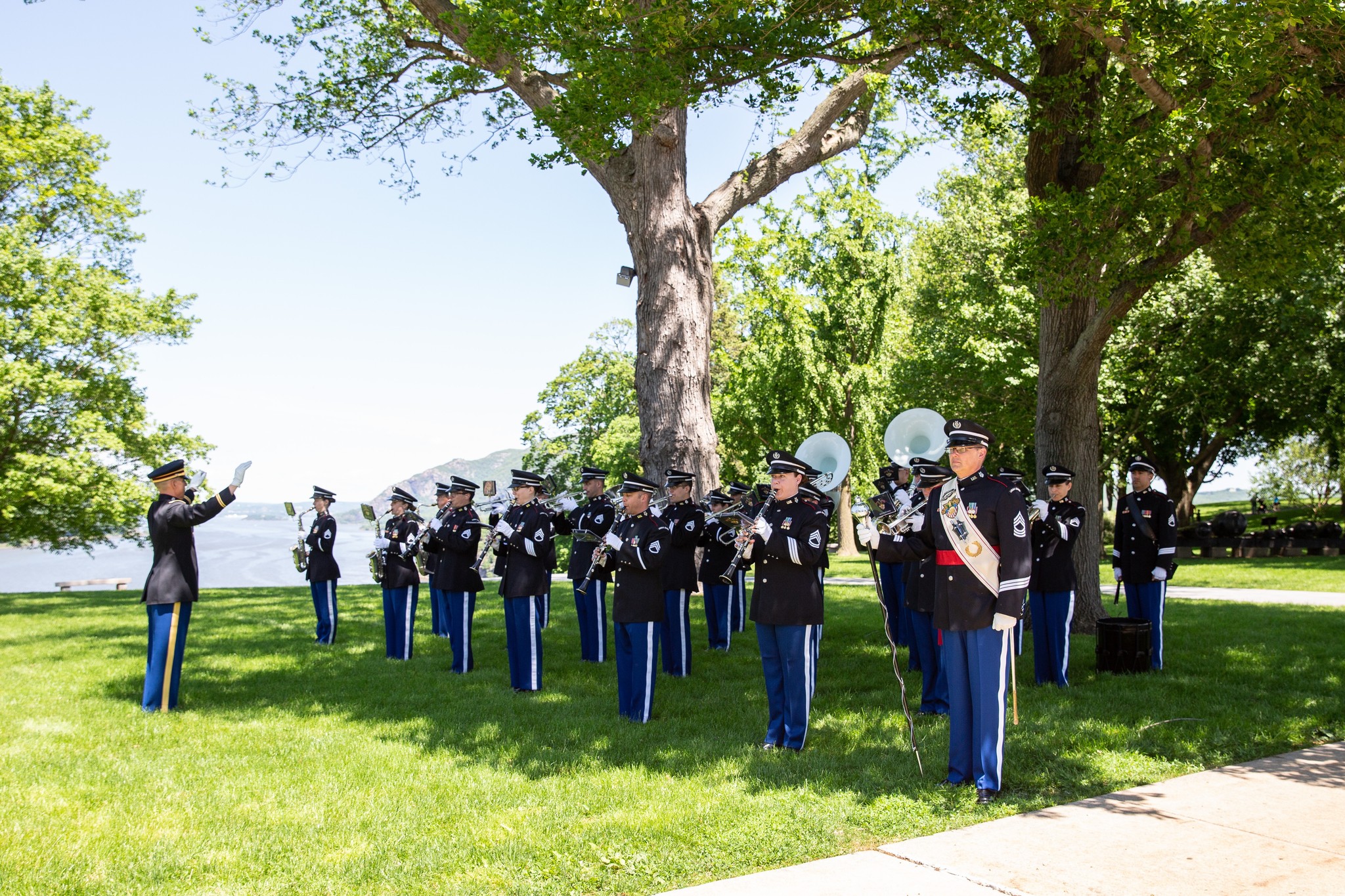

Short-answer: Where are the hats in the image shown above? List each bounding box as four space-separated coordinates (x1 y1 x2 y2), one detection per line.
309 485 337 499
706 481 751 505
997 467 1027 482
909 457 941 475
888 457 903 468
916 465 956 488
766 450 826 502
1128 455 1160 475
944 418 996 448
1042 465 1076 485
387 468 552 504
148 459 191 482
578 466 696 493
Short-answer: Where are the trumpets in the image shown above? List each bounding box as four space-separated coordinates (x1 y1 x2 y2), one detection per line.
876 497 931 535
1028 493 1056 525
472 497 515 513
616 494 673 521
538 490 590 509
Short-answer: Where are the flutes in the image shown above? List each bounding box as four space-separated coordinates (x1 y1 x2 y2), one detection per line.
470 499 517 573
575 505 626 595
397 501 455 561
719 489 779 586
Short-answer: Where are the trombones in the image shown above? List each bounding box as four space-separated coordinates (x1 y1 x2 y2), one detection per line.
704 500 744 523
605 482 625 504
718 471 834 546
868 483 918 525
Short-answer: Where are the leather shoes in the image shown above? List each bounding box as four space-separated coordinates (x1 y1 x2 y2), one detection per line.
975 788 998 804
935 779 973 788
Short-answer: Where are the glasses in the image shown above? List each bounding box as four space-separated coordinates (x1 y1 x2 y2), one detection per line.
944 446 983 454
671 485 687 490
451 491 466 497
512 486 528 491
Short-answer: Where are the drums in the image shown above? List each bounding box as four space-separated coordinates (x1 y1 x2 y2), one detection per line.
1093 618 1154 673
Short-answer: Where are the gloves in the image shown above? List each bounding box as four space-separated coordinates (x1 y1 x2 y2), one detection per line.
888 480 897 490
490 494 508 515
556 490 579 512
992 612 1017 631
905 512 924 532
231 460 252 488
894 489 912 506
188 471 206 492
898 503 912 518
1031 499 1049 521
856 516 880 549
297 531 309 540
373 535 390 548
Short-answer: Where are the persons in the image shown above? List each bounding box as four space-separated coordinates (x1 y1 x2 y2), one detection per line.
1189 504 1201 523
874 454 1032 719
418 482 452 638
138 459 253 714
297 485 342 645
1028 465 1086 690
650 469 705 680
484 470 549 694
699 465 835 659
373 485 421 661
591 471 672 725
856 418 1031 804
732 451 828 756
1112 454 1178 670
1250 492 1281 515
552 466 614 666
415 475 481 676
488 483 558 631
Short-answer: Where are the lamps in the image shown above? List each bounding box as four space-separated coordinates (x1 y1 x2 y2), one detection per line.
616 267 636 286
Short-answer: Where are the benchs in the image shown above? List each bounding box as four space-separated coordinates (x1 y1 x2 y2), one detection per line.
1176 535 1344 557
54 577 132 592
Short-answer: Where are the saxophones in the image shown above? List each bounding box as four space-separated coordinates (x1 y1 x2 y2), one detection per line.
287 506 317 572
411 501 438 576
365 508 393 584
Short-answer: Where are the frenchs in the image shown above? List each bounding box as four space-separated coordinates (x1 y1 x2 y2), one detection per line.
883 407 950 469
790 431 852 493
